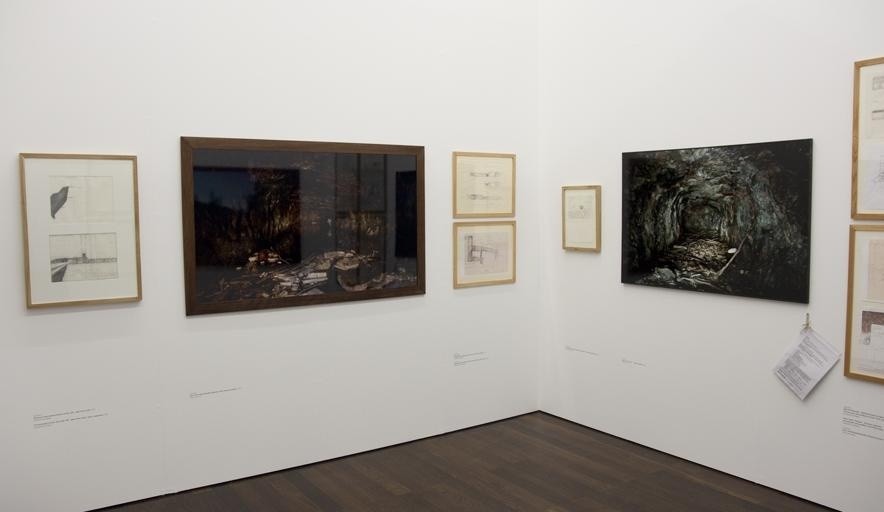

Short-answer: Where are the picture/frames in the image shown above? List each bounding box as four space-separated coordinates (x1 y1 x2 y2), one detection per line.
452 220 516 290
449 150 515 220
849 56 884 223
840 221 883 386
177 135 426 318
560 184 600 254
17 151 142 308
